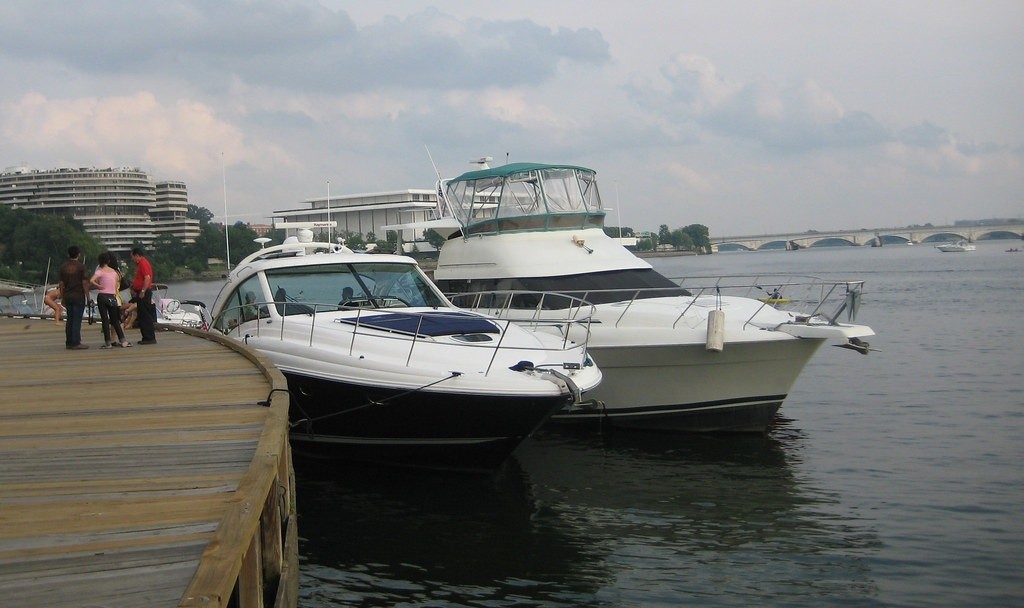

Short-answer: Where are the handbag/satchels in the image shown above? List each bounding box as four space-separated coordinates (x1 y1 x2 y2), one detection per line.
117 269 130 291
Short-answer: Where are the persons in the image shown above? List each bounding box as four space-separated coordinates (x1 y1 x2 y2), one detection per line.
272 288 288 315
767 289 782 299
44 246 156 349
228 319 237 329
336 287 360 311
242 291 260 322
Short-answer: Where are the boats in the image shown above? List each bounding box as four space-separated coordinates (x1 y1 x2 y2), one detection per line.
152 283 205 329
757 297 792 305
418 163 878 436
935 238 977 253
200 218 604 477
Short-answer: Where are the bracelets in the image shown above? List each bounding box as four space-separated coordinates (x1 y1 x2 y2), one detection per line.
141 289 146 293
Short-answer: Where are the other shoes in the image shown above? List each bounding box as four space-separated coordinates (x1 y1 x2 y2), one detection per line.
66 344 88 349
111 342 117 346
121 343 133 347
138 339 156 344
99 344 112 349
